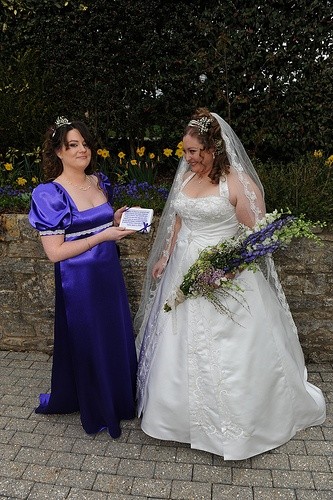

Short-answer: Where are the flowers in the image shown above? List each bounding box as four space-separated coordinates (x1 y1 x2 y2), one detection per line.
197 116 213 136
164 207 327 326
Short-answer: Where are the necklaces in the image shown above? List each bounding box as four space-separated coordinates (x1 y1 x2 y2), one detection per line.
197 169 210 184
59 174 91 191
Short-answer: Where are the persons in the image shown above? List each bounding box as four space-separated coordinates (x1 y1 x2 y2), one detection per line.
28 114 142 439
133 105 326 460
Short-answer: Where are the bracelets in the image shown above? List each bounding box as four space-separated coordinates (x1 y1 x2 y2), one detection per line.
86 237 92 250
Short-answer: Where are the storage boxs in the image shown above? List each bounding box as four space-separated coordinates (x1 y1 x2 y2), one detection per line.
119 207 154 233
